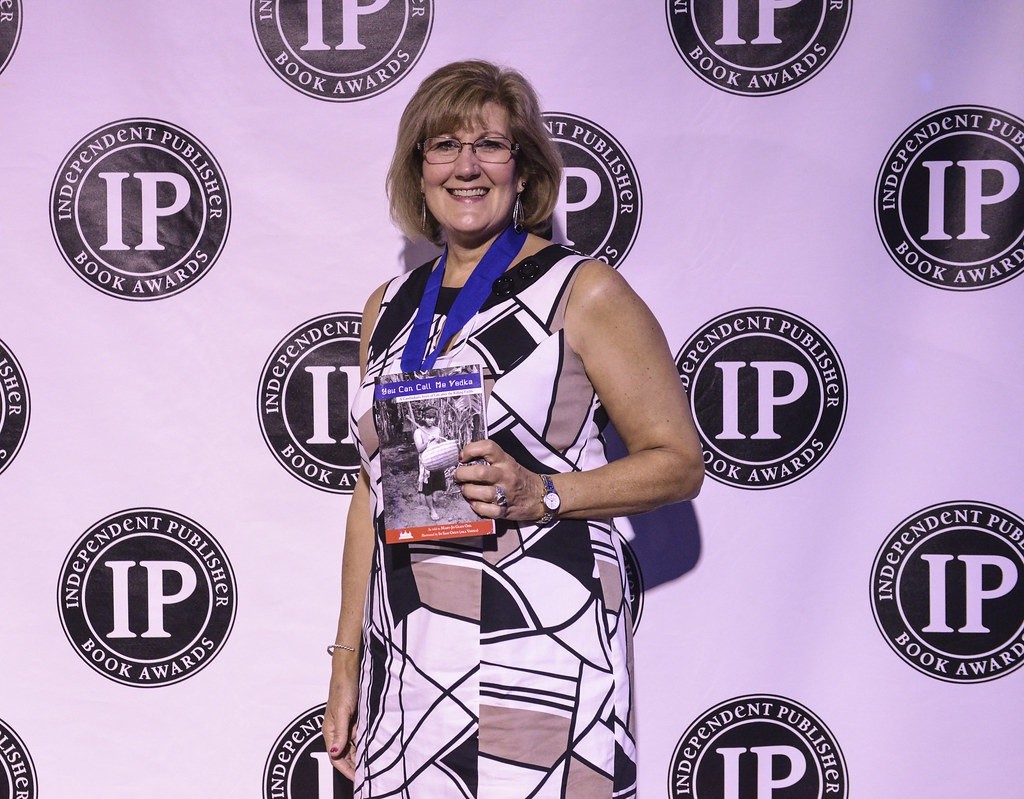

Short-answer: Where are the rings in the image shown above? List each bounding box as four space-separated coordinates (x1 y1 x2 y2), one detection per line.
493 486 507 506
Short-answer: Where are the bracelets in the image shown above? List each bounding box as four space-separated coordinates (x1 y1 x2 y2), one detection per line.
326 643 355 657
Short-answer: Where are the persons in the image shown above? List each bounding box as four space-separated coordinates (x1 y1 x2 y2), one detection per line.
321 59 707 799
412 407 446 520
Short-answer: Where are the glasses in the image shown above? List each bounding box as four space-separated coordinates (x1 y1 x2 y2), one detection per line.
416 137 519 165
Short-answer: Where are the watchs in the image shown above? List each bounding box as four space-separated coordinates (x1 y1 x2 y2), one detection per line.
535 474 561 525
